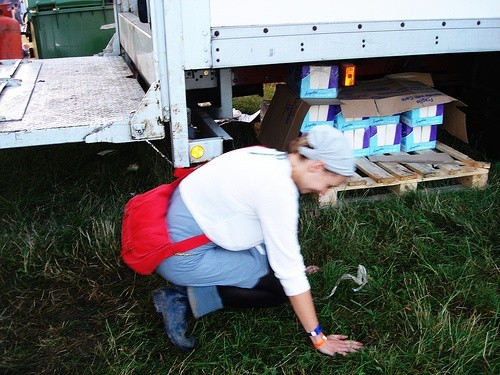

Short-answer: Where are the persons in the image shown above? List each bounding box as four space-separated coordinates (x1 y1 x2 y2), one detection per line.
152 125 364 356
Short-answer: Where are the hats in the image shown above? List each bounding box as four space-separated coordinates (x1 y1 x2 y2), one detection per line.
297 124 357 176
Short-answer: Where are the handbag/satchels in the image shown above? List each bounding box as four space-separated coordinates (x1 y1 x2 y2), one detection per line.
120 183 178 276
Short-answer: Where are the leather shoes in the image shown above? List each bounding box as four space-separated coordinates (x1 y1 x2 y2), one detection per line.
153 286 196 351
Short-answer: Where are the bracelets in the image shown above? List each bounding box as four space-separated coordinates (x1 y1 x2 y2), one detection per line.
312 333 327 347
307 324 322 337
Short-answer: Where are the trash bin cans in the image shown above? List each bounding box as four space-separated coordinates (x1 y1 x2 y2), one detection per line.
23 0 118 58
